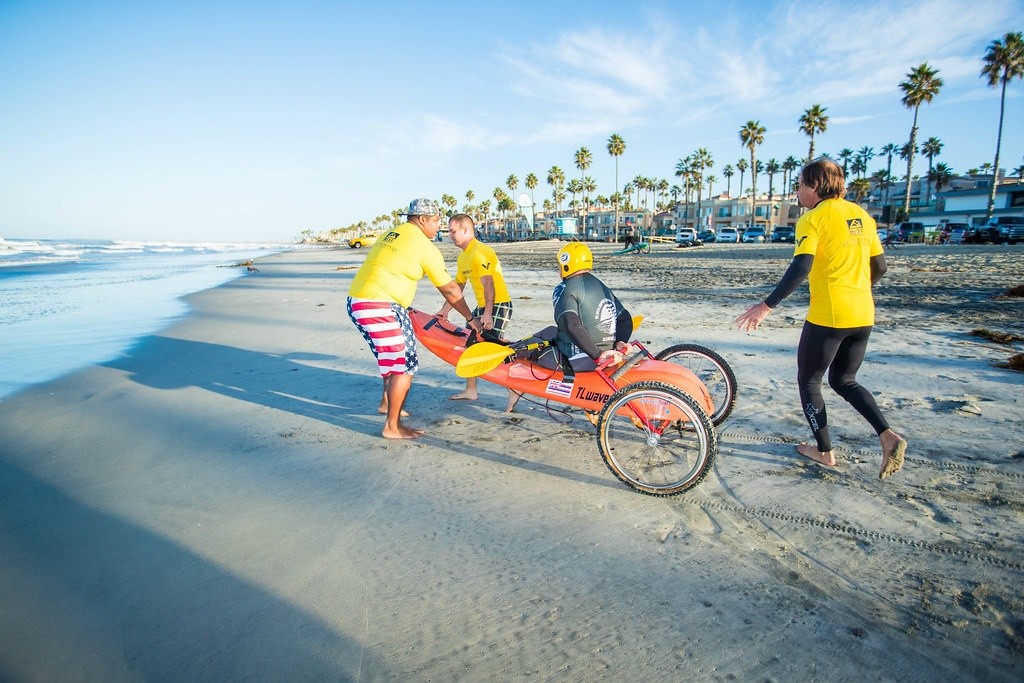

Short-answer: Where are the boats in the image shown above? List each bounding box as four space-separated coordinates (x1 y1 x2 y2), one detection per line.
408 307 715 420
614 242 648 255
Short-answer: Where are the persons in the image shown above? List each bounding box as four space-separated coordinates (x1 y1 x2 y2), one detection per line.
465 242 633 374
625 220 635 249
433 214 525 413
346 197 483 439
735 158 907 480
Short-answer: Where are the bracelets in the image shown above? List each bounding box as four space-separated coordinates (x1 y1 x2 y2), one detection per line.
466 315 474 322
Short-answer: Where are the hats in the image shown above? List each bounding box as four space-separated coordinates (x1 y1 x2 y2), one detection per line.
397 198 439 216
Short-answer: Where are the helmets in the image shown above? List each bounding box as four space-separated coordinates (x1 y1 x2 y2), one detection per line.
556 241 593 282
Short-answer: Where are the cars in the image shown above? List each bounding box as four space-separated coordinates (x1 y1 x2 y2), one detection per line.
975 215 1024 245
771 226 795 242
742 226 766 242
675 228 698 242
716 227 737 242
697 230 716 242
876 230 887 243
348 233 380 248
932 223 975 244
894 221 925 243
